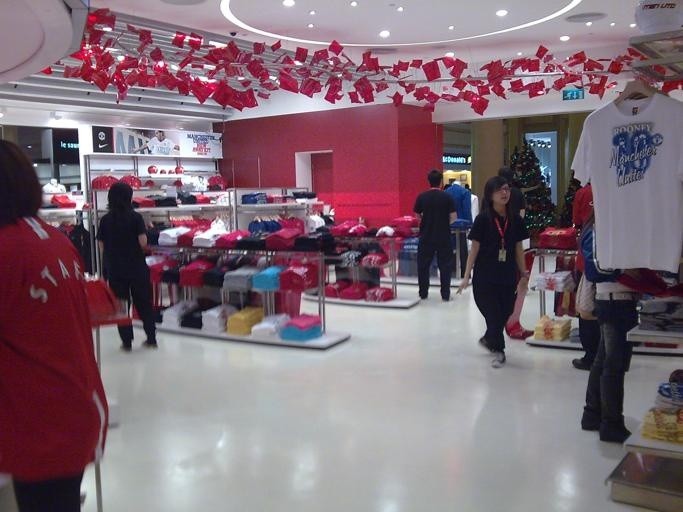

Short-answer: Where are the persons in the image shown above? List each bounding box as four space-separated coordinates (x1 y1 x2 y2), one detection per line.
131 130 180 156
411 170 457 303
93 181 158 352
444 180 472 279
0 138 110 512
41 178 66 194
454 178 530 371
578 209 632 445
571 273 600 369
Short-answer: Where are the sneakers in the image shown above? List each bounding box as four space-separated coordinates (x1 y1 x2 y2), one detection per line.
583 417 600 430
491 351 506 368
572 358 592 370
601 427 630 442
479 336 495 352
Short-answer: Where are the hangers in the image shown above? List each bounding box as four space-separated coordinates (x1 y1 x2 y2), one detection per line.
41 214 82 231
